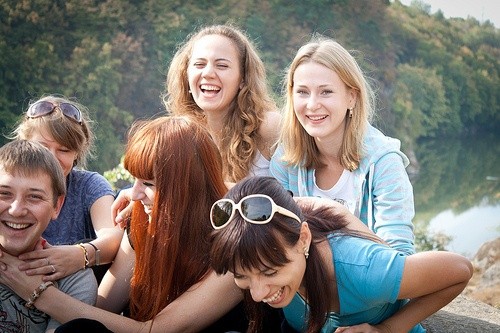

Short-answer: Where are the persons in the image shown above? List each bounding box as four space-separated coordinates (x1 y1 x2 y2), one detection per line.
110 177 474 333
5 95 125 287
270 39 416 255
0 139 98 333
163 23 282 189
0 115 386 333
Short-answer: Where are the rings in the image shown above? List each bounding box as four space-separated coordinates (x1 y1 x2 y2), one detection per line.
51 264 56 273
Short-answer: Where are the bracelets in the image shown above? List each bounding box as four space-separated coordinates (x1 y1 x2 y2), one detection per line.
89 242 100 266
78 244 89 268
25 281 54 308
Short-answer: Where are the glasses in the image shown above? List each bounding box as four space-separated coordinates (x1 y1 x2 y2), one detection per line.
27 102 83 125
210 193 301 231
